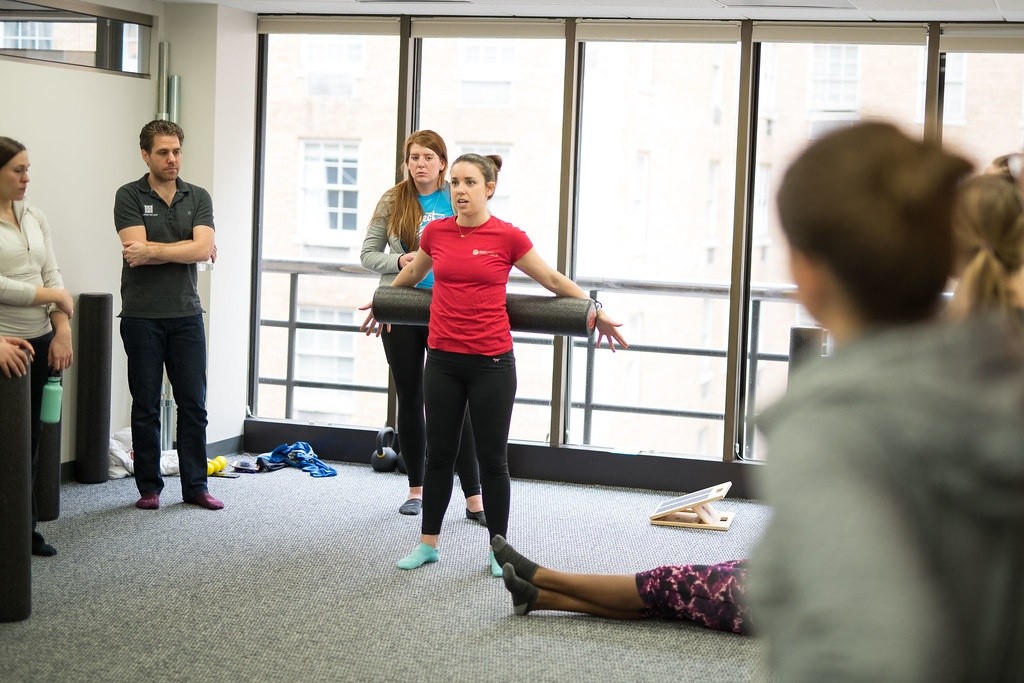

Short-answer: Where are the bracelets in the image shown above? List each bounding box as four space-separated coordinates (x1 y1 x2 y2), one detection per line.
596 300 602 310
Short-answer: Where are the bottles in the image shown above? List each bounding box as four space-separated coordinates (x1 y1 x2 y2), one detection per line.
40 368 63 423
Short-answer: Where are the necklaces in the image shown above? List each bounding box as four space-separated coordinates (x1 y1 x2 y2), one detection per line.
457 220 479 238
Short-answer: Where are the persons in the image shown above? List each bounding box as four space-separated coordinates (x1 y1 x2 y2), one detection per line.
359 152 630 577
743 121 1024 683
0 337 35 377
493 535 752 636
113 119 225 510
359 129 487 525
0 136 74 557
